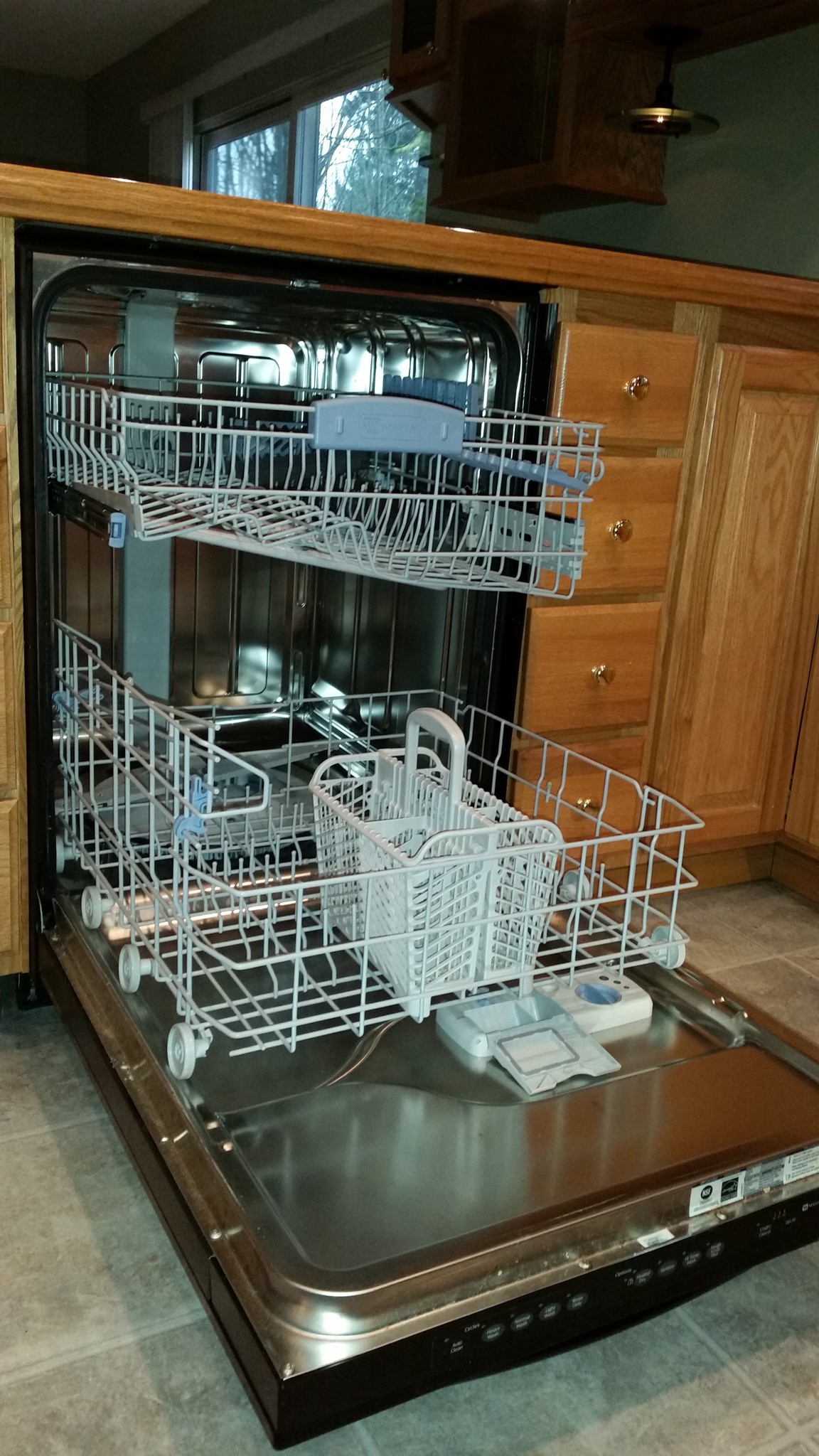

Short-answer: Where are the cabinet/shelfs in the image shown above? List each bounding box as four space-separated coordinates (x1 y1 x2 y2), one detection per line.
382 0 451 132
642 305 819 900
442 0 667 224
